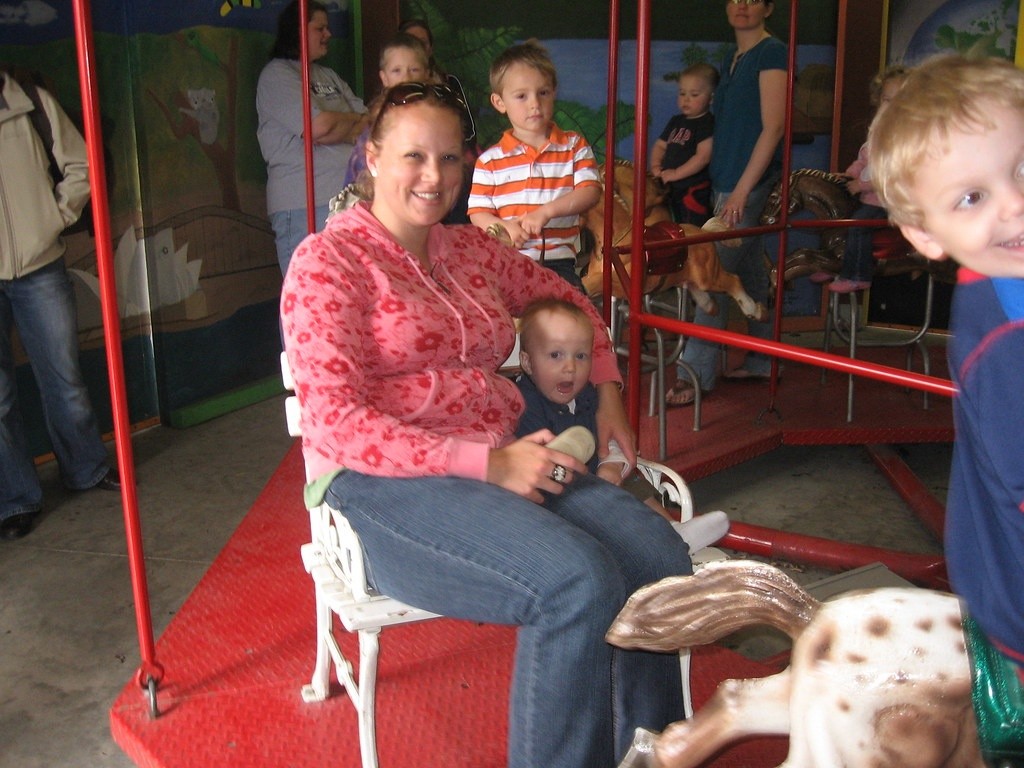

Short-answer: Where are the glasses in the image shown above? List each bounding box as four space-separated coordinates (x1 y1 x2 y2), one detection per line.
371 81 469 140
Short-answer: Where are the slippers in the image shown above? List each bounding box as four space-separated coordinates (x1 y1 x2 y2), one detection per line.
725 363 781 384
665 381 693 407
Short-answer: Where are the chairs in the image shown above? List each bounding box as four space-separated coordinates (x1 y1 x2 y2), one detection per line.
279 317 697 768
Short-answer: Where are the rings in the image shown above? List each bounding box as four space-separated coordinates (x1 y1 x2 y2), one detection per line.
552 465 566 482
733 210 739 214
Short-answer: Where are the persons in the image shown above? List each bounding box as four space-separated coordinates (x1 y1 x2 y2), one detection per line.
508 299 729 554
0 67 125 541
828 63 914 294
464 42 603 297
398 18 477 150
340 32 469 227
663 0 793 406
274 78 693 768
648 61 721 222
256 1 370 278
867 51 1024 768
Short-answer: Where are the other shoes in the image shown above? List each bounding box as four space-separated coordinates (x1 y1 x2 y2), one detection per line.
3 510 40 538
100 465 120 491
